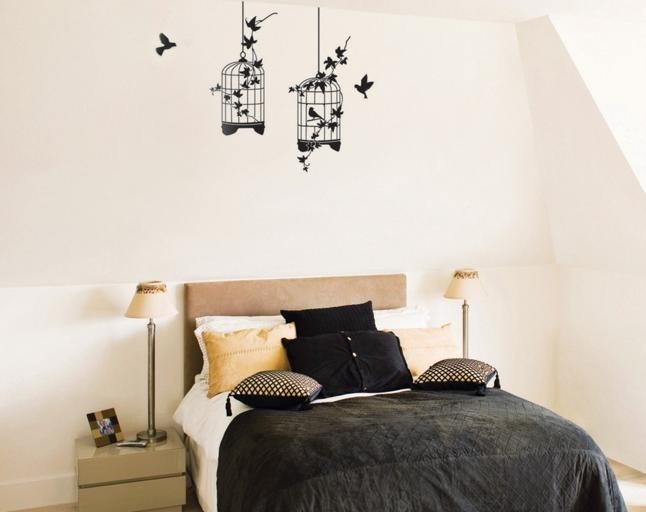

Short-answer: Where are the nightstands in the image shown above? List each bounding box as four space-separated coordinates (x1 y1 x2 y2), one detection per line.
73 427 187 512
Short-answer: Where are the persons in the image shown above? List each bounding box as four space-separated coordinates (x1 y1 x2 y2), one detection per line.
99 419 112 436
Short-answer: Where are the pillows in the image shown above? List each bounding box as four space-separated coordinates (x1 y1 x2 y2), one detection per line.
280 324 415 398
278 300 378 341
198 308 462 387
202 321 297 399
412 356 500 397
379 320 460 381
225 370 323 418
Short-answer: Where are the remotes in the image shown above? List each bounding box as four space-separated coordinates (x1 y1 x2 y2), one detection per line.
116 439 149 448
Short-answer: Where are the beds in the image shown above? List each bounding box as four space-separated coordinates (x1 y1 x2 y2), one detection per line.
172 273 628 512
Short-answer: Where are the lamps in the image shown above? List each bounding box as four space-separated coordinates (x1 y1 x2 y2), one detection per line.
444 268 491 357
124 279 180 444
